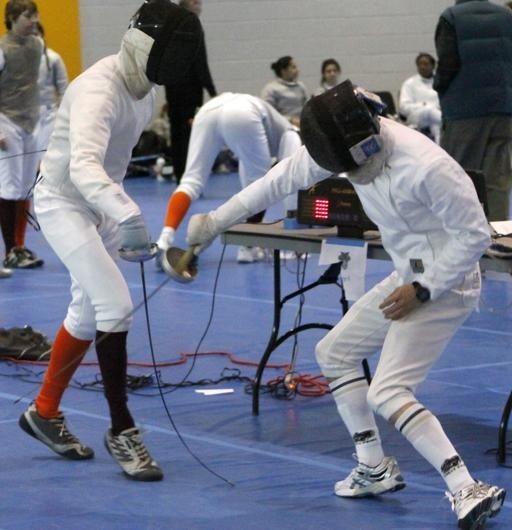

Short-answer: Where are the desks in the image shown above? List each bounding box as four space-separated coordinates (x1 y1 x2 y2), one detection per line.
218 222 511 470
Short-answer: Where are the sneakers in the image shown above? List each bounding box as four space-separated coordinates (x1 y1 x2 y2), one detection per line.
155 242 170 273
237 245 272 265
18 402 94 461
445 479 506 529
329 456 406 498
103 428 164 481
2 246 45 269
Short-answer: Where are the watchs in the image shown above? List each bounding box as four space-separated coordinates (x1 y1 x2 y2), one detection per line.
412 280 431 303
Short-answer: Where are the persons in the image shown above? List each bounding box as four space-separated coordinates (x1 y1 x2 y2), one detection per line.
431 0 512 222
182 79 507 529
0 1 45 280
30 21 70 164
258 52 311 126
148 101 172 149
151 88 302 272
14 1 206 483
307 58 343 99
397 51 444 143
165 0 218 186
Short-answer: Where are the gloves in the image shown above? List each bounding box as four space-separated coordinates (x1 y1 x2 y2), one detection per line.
184 212 221 258
118 214 154 260
